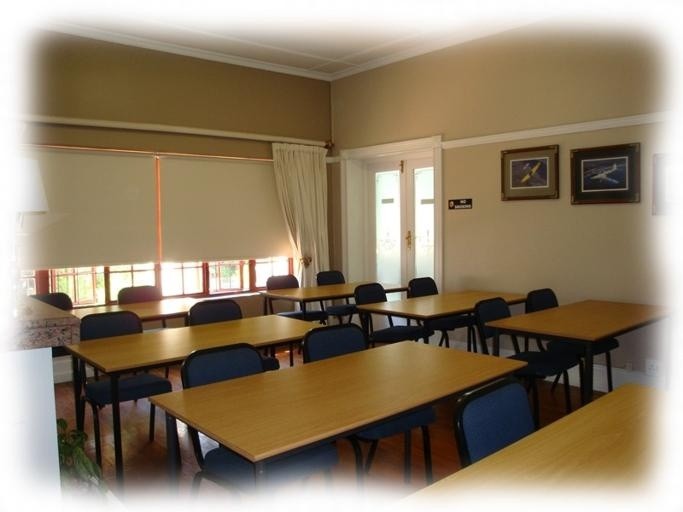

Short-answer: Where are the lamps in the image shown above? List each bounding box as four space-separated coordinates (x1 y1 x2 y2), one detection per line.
9 156 49 322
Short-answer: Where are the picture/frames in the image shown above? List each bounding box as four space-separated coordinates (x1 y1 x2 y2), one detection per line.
569 140 641 205
498 145 559 200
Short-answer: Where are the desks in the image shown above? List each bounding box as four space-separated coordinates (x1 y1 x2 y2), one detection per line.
148 339 531 492
257 280 361 357
61 314 326 482
354 291 525 347
486 297 663 407
423 385 657 492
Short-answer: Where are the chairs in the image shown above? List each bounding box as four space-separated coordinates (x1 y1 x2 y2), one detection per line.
524 288 620 408
74 311 182 471
408 278 478 352
475 297 584 427
452 372 541 475
316 270 364 327
299 323 439 487
35 293 100 407
354 281 433 347
264 275 328 358
120 290 171 408
177 343 342 491
186 299 279 370
